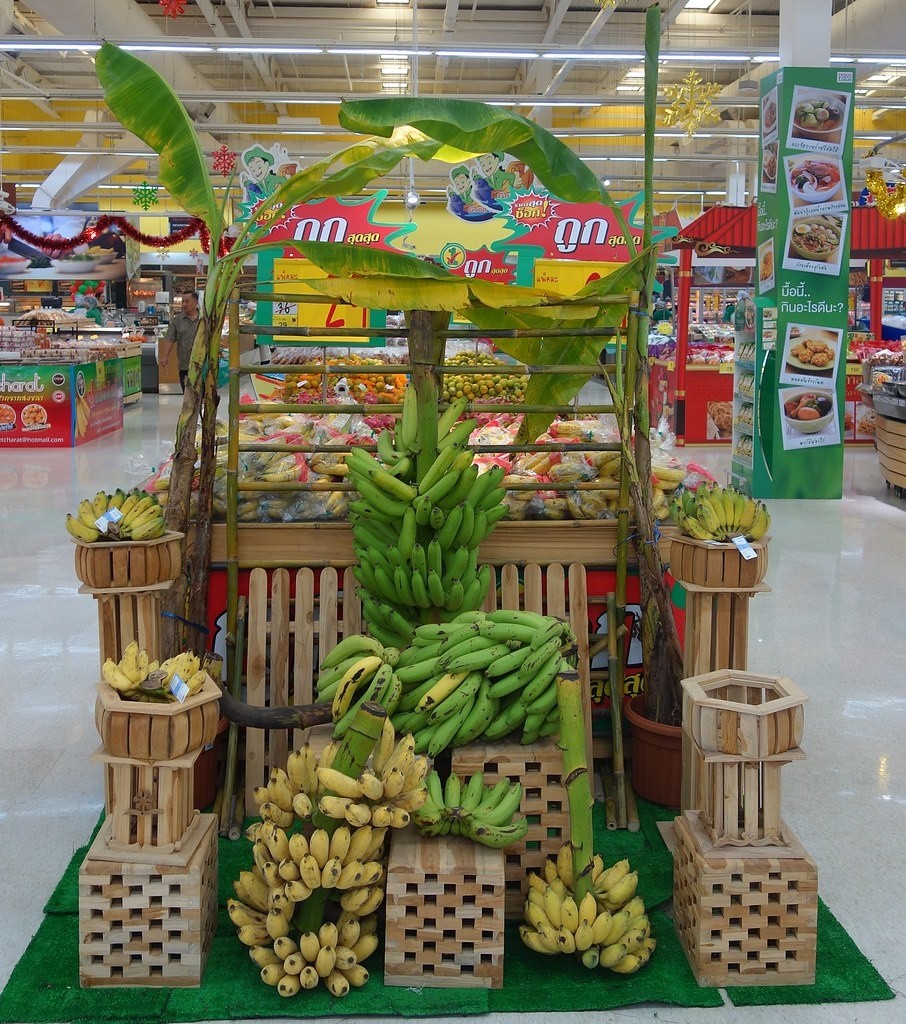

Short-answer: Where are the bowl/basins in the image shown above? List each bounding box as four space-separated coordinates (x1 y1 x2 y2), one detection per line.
790 219 841 260
50 259 99 272
784 391 834 433
793 93 846 133
790 159 840 202
760 245 773 282
89 252 118 264
0 260 31 273
763 97 776 133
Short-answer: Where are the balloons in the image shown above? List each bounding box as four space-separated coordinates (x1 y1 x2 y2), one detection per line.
70 279 106 302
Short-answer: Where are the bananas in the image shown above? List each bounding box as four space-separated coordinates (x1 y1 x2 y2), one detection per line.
68 381 769 995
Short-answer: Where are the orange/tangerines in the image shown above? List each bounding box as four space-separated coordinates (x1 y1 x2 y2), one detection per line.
284 352 528 405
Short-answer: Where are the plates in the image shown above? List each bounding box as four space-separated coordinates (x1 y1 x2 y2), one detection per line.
786 336 835 370
0 404 16 425
21 404 47 427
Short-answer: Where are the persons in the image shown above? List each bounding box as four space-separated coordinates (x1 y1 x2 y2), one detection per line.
0 215 87 261
96 291 104 305
83 296 102 325
161 290 200 395
247 302 257 315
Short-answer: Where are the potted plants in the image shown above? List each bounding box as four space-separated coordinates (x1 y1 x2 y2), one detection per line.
335 91 684 811
94 39 588 812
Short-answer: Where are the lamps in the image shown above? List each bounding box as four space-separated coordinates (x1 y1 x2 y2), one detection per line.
405 190 420 211
